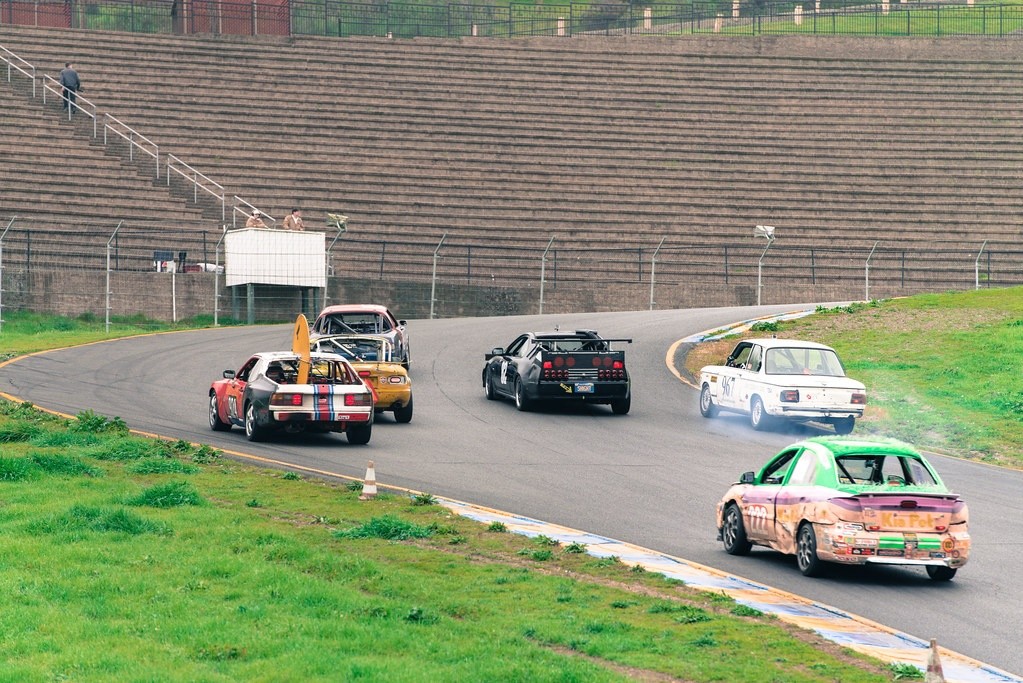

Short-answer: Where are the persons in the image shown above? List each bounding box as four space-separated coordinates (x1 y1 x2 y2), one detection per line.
245 209 268 228
59 62 81 114
282 208 305 231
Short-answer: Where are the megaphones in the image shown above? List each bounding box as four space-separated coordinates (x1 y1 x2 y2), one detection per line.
326 214 349 229
754 226 776 238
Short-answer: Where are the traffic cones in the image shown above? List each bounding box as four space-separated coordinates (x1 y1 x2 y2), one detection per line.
358 462 380 500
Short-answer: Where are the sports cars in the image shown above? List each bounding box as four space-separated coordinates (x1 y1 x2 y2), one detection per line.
482 324 633 414
209 304 414 445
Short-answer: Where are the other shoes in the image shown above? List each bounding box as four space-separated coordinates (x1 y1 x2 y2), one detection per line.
72 108 76 114
63 107 68 112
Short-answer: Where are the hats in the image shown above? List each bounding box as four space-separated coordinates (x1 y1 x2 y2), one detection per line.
252 210 260 215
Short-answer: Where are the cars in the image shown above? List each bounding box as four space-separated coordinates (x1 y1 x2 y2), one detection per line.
715 434 970 582
699 339 868 434
